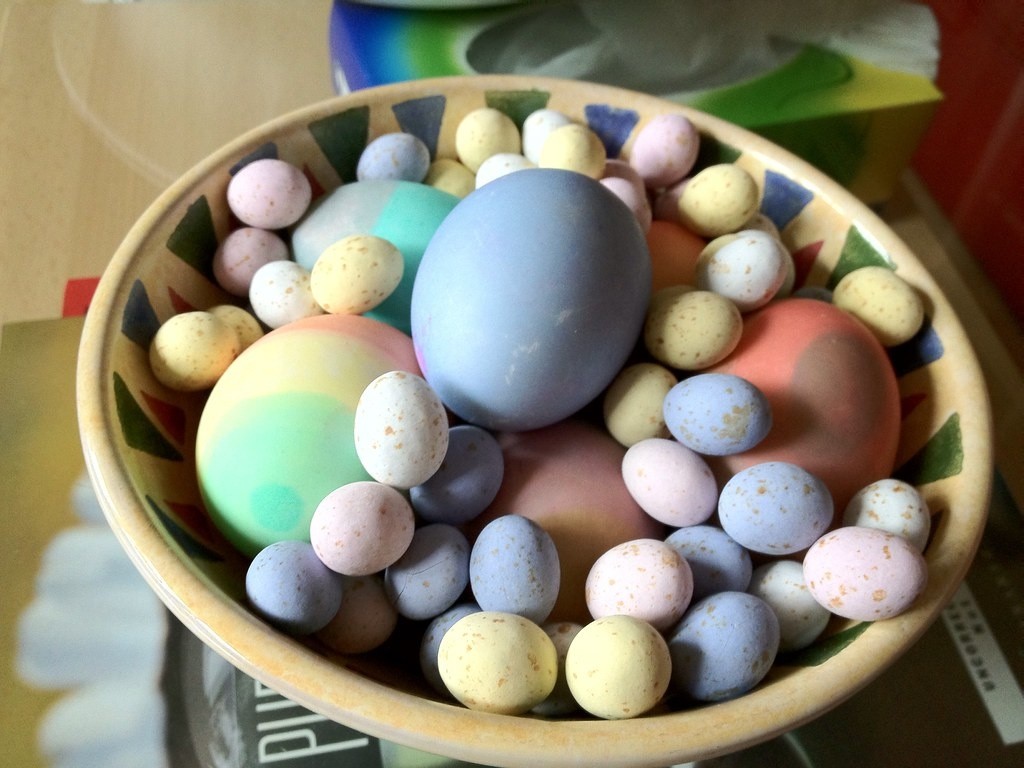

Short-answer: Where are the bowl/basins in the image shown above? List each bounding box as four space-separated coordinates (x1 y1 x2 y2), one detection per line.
74 74 991 768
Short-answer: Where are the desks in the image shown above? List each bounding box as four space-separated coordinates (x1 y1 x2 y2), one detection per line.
0 215 1024 768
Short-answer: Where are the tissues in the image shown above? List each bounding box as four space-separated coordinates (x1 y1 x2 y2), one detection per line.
330 1 945 205
